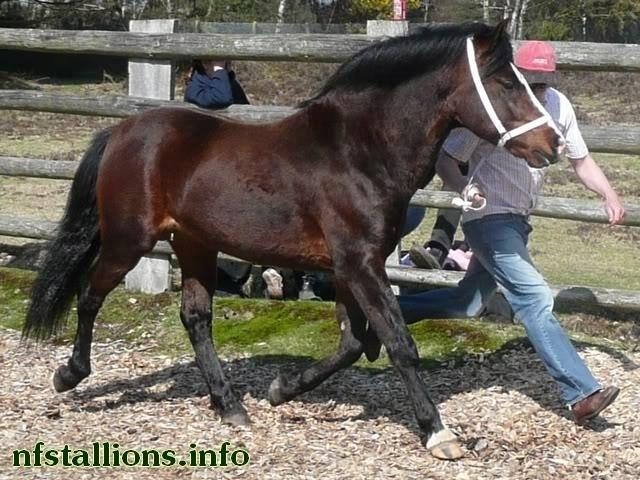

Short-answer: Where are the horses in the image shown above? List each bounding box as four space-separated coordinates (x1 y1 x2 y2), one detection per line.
17 12 567 464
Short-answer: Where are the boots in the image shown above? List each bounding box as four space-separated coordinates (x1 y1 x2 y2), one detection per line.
409 244 447 269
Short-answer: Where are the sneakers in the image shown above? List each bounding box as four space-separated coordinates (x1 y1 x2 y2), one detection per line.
262 268 283 299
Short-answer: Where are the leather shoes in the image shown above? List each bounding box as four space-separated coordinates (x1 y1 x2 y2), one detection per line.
364 320 382 362
572 386 620 425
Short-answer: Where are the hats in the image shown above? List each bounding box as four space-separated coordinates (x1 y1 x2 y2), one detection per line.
513 40 556 71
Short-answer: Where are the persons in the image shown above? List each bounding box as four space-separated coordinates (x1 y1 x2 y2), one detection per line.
364 40 625 425
184 59 285 299
